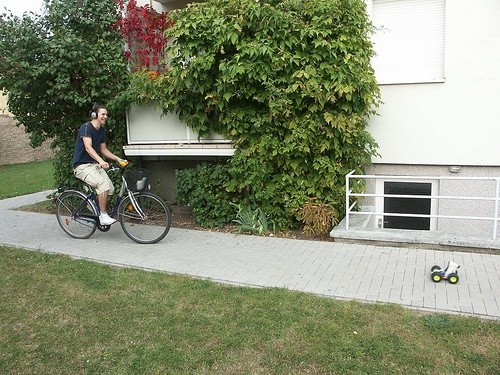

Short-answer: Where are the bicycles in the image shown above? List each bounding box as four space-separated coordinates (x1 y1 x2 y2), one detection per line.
54 160 172 246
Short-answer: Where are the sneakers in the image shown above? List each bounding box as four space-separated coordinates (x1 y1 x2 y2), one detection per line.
87 199 96 213
99 213 117 225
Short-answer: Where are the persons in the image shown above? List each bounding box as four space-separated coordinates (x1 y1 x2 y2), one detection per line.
73 103 129 226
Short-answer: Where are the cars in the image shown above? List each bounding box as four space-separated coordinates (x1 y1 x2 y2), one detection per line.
430 259 461 284
